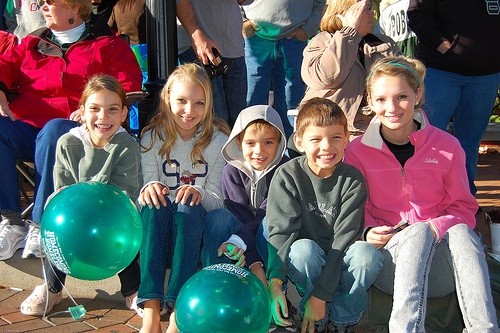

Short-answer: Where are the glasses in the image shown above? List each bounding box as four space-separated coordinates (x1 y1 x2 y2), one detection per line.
38 0 53 7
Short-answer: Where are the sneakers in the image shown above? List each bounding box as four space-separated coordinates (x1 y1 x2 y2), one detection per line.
0 219 27 261
21 221 47 260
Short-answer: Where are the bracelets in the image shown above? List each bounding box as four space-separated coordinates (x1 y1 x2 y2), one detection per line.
243 19 249 23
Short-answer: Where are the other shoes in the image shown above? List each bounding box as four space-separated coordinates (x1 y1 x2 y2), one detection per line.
298 321 326 333
328 322 354 333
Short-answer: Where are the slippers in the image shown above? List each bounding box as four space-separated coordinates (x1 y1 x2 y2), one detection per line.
125 290 168 319
20 283 63 316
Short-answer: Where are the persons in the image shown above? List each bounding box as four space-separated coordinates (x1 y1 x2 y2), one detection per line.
176 0 254 128
406 0 500 196
300 0 403 142
343 55 499 333
20 74 143 316
0 0 148 260
240 0 327 139
256 97 383 333
373 0 420 61
135 62 228 333
221 105 291 286
